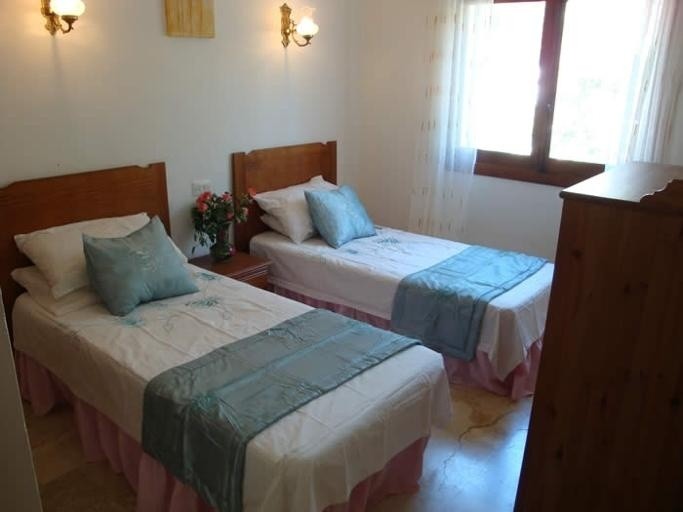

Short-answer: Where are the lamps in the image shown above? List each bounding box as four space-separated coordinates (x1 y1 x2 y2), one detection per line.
38 0 86 34
279 1 316 52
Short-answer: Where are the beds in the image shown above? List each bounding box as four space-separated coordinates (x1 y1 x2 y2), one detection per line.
3 158 458 512
232 139 557 403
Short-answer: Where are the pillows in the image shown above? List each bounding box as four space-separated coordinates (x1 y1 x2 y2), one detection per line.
11 261 99 314
12 210 187 299
303 181 377 247
255 174 339 243
83 214 200 318
259 212 319 244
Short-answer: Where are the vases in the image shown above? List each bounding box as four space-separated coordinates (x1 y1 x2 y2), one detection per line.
209 221 235 262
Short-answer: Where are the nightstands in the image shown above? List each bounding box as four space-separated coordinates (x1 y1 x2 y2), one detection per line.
187 247 274 291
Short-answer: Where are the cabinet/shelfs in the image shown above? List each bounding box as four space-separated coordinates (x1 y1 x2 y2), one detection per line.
505 163 680 511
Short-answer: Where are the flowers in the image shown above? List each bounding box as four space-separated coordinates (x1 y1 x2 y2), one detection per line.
189 187 257 253
